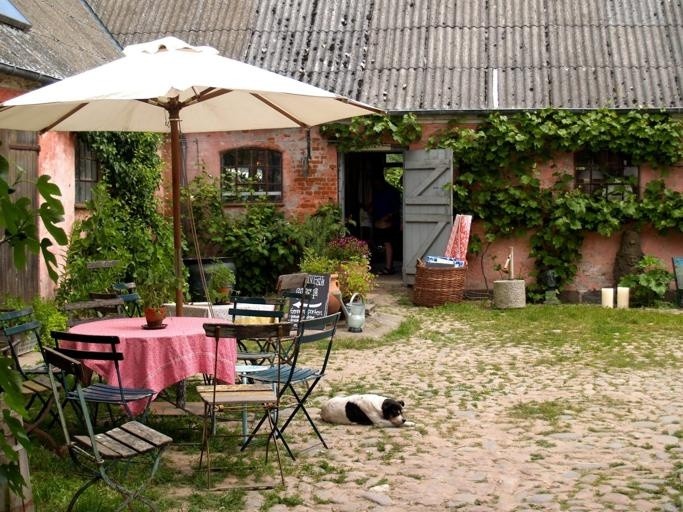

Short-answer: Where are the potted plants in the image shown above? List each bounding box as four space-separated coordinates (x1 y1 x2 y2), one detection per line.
297 247 377 320
180 171 235 302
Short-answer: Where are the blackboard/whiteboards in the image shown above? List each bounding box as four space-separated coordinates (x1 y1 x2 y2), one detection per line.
287 274 330 330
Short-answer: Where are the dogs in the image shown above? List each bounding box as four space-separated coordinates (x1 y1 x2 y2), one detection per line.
319 392 416 428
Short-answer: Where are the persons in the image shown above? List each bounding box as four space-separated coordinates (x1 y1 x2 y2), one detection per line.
369 173 401 275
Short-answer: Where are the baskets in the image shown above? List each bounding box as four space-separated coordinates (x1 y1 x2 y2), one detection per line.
412 258 468 308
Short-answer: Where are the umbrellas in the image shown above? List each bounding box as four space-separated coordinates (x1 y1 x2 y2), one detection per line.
0 35 388 408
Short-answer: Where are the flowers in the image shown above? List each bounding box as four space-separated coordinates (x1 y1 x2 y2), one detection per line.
329 236 369 259
